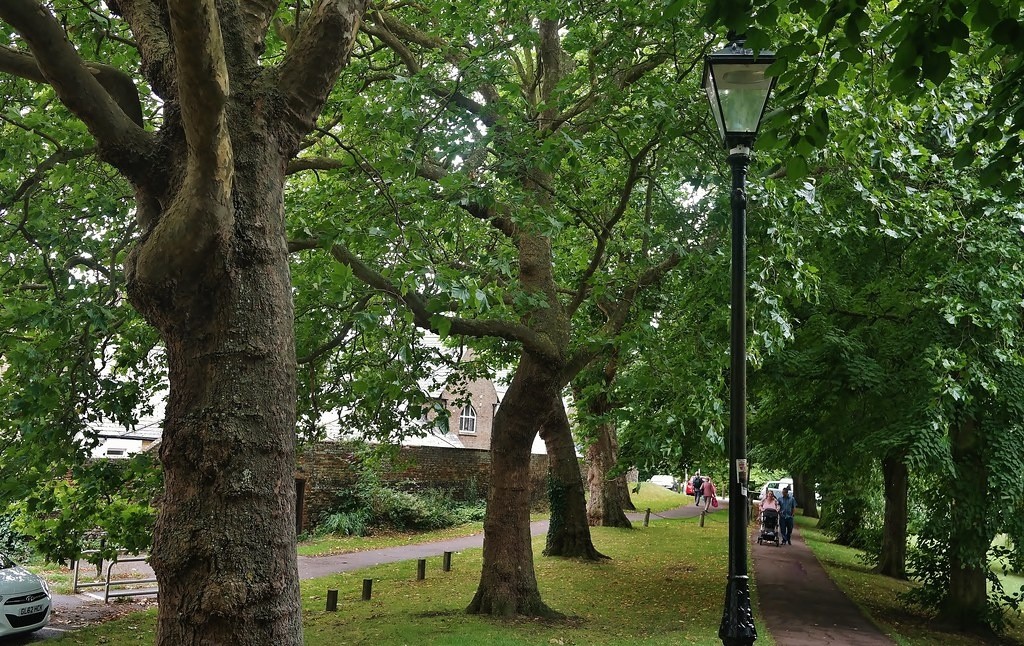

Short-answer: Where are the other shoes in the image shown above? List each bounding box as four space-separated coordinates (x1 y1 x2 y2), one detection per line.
788 540 791 545
781 543 785 545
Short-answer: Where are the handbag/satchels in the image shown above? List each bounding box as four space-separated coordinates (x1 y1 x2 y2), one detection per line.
700 484 704 495
712 497 718 508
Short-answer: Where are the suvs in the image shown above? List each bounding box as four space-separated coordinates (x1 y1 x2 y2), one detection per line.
759 481 794 502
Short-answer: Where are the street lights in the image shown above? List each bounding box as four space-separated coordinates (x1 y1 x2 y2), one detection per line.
700 39 788 644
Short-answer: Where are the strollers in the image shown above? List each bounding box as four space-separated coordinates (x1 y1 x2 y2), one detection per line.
757 508 780 546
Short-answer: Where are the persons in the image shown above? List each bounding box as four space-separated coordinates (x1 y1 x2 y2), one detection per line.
777 487 798 545
759 490 780 543
699 477 715 512
692 471 704 506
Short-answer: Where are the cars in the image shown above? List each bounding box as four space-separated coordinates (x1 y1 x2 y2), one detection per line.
688 475 710 496
0 550 51 638
648 475 678 492
